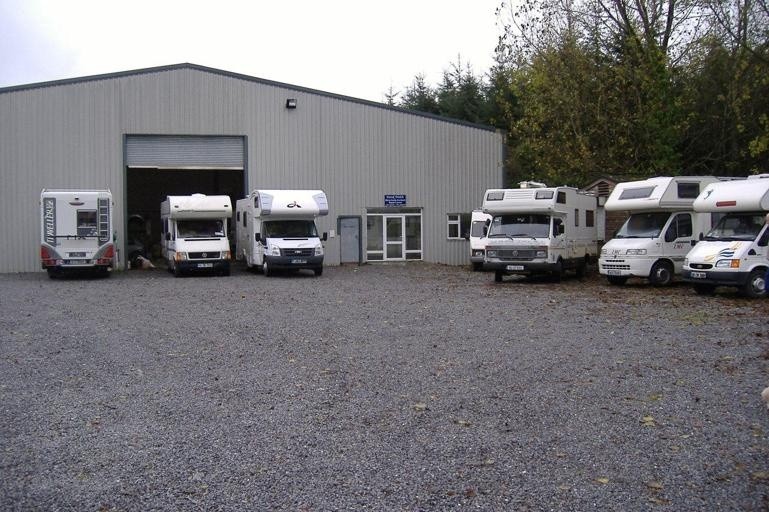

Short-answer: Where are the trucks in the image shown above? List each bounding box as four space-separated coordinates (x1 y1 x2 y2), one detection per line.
37 187 117 276
234 188 332 276
157 191 233 278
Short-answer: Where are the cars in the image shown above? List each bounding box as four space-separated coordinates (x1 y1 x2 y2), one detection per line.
128 214 148 266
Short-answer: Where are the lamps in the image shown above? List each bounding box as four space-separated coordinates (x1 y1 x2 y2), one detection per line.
286 98 297 109
